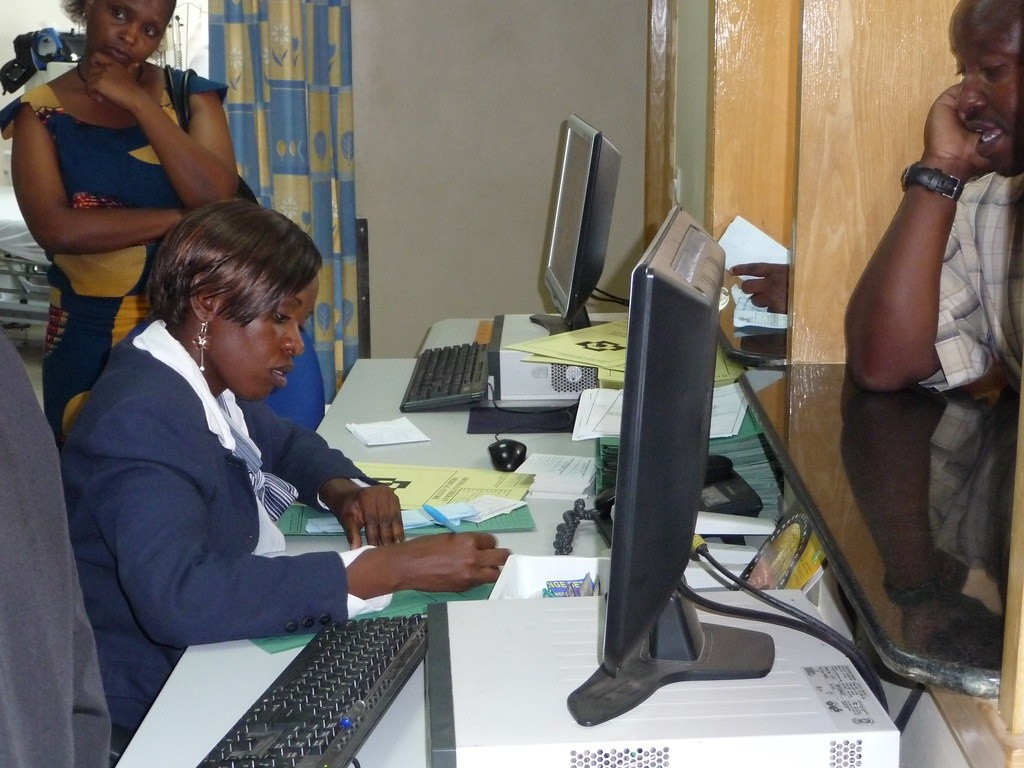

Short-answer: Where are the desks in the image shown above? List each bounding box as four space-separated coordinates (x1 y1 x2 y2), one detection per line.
115 359 757 767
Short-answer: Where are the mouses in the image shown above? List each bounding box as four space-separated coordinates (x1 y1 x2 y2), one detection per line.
488 439 527 472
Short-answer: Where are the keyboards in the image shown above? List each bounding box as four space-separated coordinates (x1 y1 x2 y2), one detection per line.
196 613 429 768
399 341 489 412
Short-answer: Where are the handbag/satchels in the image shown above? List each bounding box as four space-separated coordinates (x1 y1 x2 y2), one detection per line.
166 66 260 203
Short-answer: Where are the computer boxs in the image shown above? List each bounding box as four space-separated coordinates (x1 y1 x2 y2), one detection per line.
488 311 628 399
426 587 901 768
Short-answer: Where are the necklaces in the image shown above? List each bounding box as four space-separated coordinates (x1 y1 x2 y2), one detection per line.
78 56 143 86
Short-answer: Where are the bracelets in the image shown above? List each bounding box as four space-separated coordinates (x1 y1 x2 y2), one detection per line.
900 162 964 201
882 577 934 606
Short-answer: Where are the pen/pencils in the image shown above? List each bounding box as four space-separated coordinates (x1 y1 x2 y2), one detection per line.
421 504 459 535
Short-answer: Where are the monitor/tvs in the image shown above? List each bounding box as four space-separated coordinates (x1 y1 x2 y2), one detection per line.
565 203 775 727
528 115 622 337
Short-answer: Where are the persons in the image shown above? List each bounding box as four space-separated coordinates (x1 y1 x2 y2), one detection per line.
843 0 1024 391
0 0 239 453
730 262 788 314
843 392 1020 669
0 327 112 768
59 199 510 733
